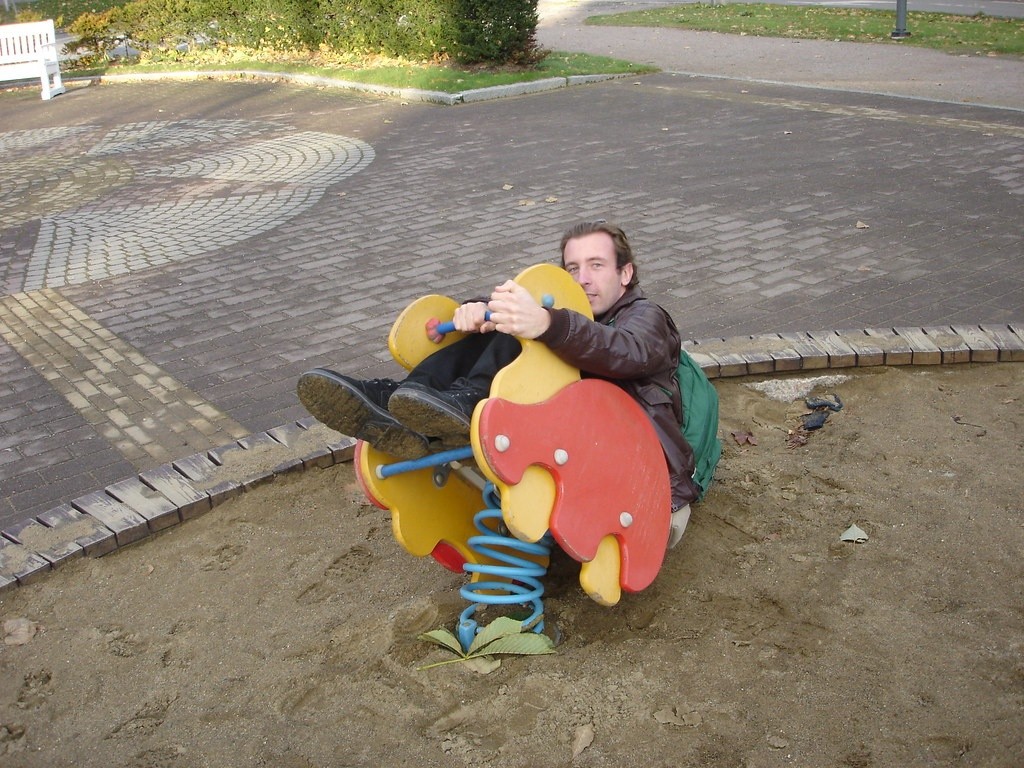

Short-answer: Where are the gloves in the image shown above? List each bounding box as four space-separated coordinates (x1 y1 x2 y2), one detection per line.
800 410 830 431
805 393 843 411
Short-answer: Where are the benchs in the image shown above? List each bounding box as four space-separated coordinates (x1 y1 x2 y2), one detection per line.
0 19 66 100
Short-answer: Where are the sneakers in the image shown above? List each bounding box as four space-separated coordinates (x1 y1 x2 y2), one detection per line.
387 377 491 446
296 368 442 459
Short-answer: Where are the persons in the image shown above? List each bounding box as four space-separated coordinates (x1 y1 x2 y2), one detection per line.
297 218 704 511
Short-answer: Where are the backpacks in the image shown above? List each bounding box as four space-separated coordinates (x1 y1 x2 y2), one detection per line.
607 302 722 503
